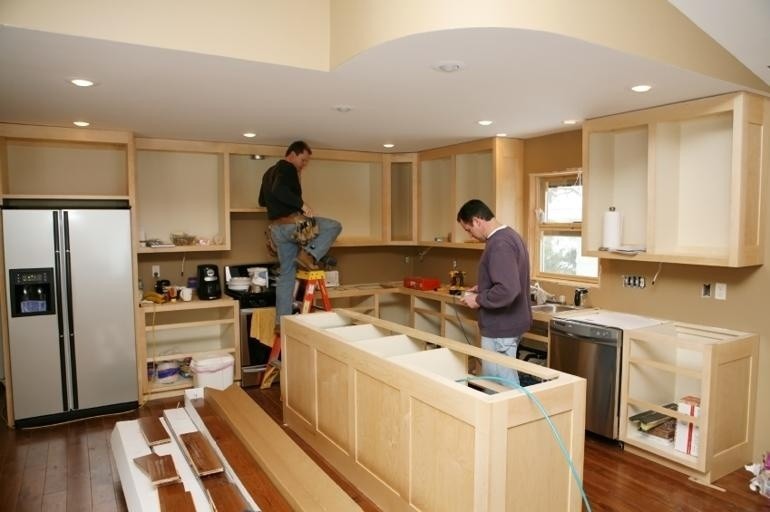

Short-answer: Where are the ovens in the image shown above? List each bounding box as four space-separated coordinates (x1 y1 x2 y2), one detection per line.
241 307 281 389
549 319 622 442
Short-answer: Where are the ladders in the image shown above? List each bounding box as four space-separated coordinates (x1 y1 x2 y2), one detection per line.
260 270 332 402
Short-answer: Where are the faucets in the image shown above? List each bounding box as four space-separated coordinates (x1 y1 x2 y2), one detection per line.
574 288 588 306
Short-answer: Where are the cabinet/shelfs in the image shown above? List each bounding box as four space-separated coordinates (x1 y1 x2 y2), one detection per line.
378 286 442 351
300 148 387 248
442 294 551 394
228 143 300 214
133 137 230 254
618 321 759 484
387 154 416 246
581 91 769 268
418 136 523 253
314 288 379 325
138 291 241 402
1 122 134 201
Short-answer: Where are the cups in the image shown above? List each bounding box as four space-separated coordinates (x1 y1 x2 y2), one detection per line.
179 287 193 301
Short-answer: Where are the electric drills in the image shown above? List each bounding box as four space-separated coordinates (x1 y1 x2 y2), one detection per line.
448 270 467 295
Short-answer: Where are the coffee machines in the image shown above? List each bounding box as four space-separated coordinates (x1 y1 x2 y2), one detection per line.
195 263 222 301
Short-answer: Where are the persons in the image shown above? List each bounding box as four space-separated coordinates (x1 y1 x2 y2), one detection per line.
256 138 342 335
451 196 533 400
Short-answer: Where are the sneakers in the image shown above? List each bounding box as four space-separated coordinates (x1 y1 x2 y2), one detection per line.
294 253 319 270
273 326 281 335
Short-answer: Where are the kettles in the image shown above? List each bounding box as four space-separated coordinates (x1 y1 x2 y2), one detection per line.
574 285 589 309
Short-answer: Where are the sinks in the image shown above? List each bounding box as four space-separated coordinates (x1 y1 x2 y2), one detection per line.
529 304 601 314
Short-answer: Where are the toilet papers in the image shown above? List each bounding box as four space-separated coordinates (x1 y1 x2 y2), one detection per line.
602 210 625 248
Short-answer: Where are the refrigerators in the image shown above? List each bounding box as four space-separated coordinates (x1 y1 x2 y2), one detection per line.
1 194 141 437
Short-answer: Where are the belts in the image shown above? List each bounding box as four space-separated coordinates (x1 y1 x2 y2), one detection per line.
271 210 301 224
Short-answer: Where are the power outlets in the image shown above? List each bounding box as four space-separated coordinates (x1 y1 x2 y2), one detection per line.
715 283 727 302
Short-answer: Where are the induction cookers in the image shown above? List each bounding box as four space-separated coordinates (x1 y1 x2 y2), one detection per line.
226 287 304 305
553 309 662 330
